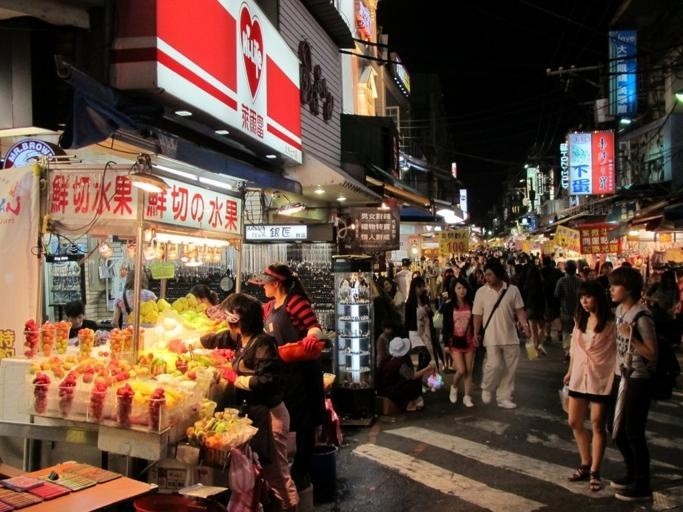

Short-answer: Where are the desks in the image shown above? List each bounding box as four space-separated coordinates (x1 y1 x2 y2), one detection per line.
0 460 157 512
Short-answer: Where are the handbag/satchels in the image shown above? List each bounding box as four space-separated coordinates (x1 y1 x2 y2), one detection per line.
474 347 486 366
451 334 469 349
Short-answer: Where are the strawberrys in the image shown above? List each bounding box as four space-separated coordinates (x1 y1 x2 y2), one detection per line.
116 384 134 426
89 382 107 420
58 375 76 416
24 319 39 359
149 387 165 430
32 371 50 414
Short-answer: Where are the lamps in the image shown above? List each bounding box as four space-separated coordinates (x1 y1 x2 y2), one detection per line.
131 173 169 193
335 217 355 239
606 204 618 223
274 191 305 215
675 89 683 102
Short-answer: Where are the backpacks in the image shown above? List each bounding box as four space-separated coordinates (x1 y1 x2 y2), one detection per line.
631 310 681 401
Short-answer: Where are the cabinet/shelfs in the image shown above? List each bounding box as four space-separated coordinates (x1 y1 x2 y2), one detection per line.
332 257 376 420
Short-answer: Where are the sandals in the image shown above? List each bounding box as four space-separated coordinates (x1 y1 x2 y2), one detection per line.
589 471 603 492
568 463 590 481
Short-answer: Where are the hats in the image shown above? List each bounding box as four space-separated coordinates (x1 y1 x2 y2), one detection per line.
401 258 412 266
205 303 241 323
248 267 286 285
388 337 410 358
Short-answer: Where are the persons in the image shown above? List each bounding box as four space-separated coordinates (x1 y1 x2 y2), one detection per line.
112 269 158 330
563 280 615 492
189 285 219 311
64 300 99 340
554 258 682 433
185 292 301 511
609 265 658 500
375 243 567 417
261 263 337 511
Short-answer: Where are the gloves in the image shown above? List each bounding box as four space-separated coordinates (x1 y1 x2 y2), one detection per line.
216 366 254 391
297 327 323 353
168 337 205 355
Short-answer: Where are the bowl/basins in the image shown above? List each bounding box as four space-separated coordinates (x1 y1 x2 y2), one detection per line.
134 493 198 511
279 342 325 362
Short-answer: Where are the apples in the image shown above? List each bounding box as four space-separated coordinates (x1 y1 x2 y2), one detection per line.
129 292 207 323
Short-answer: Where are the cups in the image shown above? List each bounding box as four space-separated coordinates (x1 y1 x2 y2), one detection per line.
115 394 134 427
56 385 77 417
194 370 213 393
145 397 165 428
31 383 50 414
525 344 535 361
87 389 106 420
20 325 151 383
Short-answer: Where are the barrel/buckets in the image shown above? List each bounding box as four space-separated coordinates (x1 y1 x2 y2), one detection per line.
313 444 339 506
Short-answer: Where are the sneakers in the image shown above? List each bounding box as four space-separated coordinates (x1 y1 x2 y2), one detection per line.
414 396 424 407
481 390 492 404
610 474 633 488
614 488 652 502
497 400 517 409
463 395 474 408
449 384 458 403
